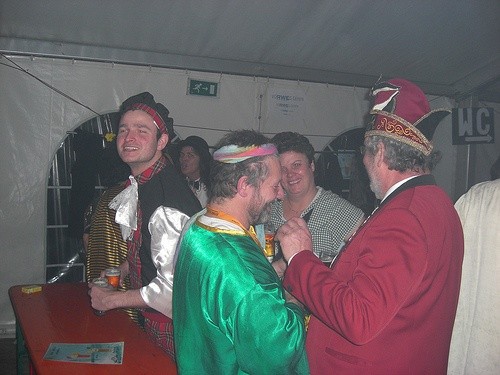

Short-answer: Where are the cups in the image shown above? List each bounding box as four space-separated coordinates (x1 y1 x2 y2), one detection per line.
106 268 121 288
92 277 108 316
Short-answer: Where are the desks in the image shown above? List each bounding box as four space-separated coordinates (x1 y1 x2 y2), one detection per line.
7 280 178 375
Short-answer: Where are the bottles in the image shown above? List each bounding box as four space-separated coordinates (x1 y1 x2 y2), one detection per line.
264 221 275 264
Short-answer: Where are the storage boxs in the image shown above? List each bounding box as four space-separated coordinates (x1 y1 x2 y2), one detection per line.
20 284 42 294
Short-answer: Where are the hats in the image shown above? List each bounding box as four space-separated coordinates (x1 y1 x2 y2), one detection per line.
167 135 217 172
120 92 174 151
364 73 452 156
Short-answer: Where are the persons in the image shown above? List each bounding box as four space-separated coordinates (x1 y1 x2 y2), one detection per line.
271 131 366 262
172 131 311 375
179 135 210 208
273 79 464 375
82 91 203 359
446 179 500 375
